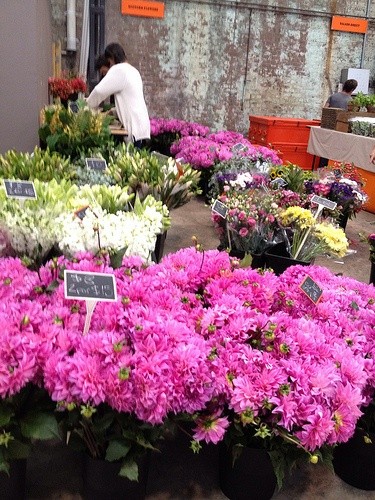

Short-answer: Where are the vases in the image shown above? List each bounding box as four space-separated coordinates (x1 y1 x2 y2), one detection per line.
216 243 264 270
0 458 26 500
80 458 145 500
332 413 375 492
264 242 312 276
218 434 277 500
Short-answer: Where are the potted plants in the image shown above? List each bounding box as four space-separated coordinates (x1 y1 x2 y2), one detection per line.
357 91 375 112
347 97 360 112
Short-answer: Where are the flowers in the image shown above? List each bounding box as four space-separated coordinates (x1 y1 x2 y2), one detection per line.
0 76 375 469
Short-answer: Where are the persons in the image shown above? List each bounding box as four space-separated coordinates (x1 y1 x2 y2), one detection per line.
368 148 375 165
325 78 358 110
81 42 152 150
